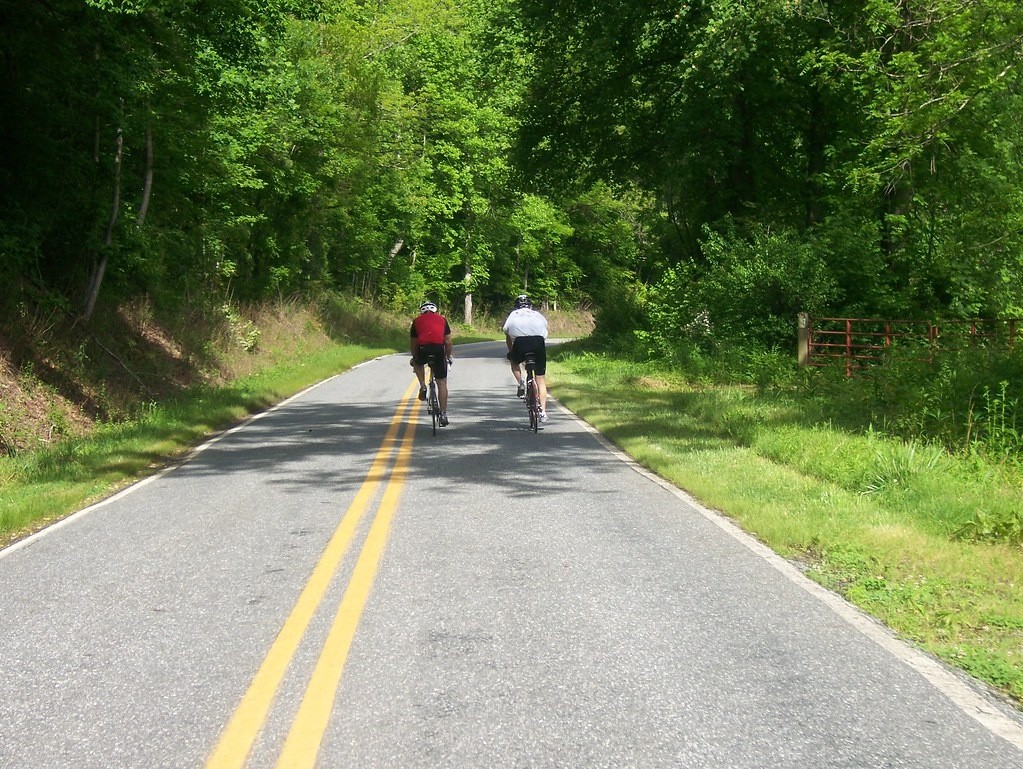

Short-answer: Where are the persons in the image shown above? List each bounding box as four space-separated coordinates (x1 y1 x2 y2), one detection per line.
410 302 452 425
503 295 549 423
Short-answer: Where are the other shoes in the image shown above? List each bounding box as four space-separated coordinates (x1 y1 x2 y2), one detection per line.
419 386 427 401
539 413 548 423
441 415 449 425
517 380 525 397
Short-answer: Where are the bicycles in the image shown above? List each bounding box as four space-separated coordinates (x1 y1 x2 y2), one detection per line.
505 352 544 434
410 356 452 435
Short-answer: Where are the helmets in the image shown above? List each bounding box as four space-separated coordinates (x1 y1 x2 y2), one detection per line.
420 302 437 314
514 294 532 309
